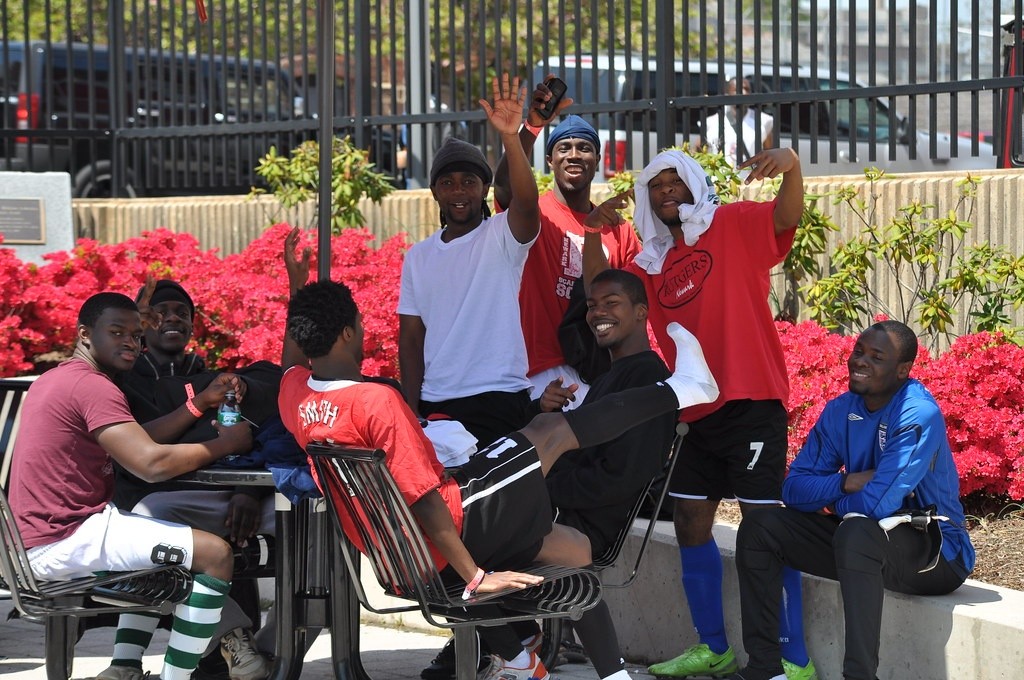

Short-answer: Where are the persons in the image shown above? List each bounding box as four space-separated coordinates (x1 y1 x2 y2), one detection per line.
694 77 774 169
108 274 285 679
431 269 679 680
718 321 975 679
391 71 646 680
7 292 254 679
581 145 816 679
396 95 466 190
277 224 637 680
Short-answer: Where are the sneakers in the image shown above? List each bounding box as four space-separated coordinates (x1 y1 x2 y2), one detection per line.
217 627 266 680
483 630 552 680
95 666 151 680
781 657 819 680
421 635 457 680
648 644 740 676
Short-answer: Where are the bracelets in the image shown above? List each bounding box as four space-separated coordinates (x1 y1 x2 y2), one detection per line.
184 384 204 418
823 507 832 514
461 566 484 600
523 117 545 139
581 223 604 234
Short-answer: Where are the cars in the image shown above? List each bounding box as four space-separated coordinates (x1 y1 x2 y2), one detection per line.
297 74 471 189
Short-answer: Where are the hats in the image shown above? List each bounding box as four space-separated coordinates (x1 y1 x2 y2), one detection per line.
546 114 602 148
136 279 193 305
429 136 493 229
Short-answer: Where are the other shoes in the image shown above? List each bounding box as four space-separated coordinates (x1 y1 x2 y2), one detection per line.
554 636 588 668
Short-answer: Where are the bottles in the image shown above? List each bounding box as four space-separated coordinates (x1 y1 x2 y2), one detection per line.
217 388 241 461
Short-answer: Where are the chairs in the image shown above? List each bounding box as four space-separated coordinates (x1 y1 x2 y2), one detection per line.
0 482 194 680
569 419 688 591
306 443 603 680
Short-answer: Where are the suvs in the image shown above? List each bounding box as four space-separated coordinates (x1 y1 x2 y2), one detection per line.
502 50 1001 173
0 39 399 199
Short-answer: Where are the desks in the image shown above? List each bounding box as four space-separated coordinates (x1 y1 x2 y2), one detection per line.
41 465 562 680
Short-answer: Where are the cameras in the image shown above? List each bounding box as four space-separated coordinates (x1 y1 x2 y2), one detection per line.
535 78 567 120
